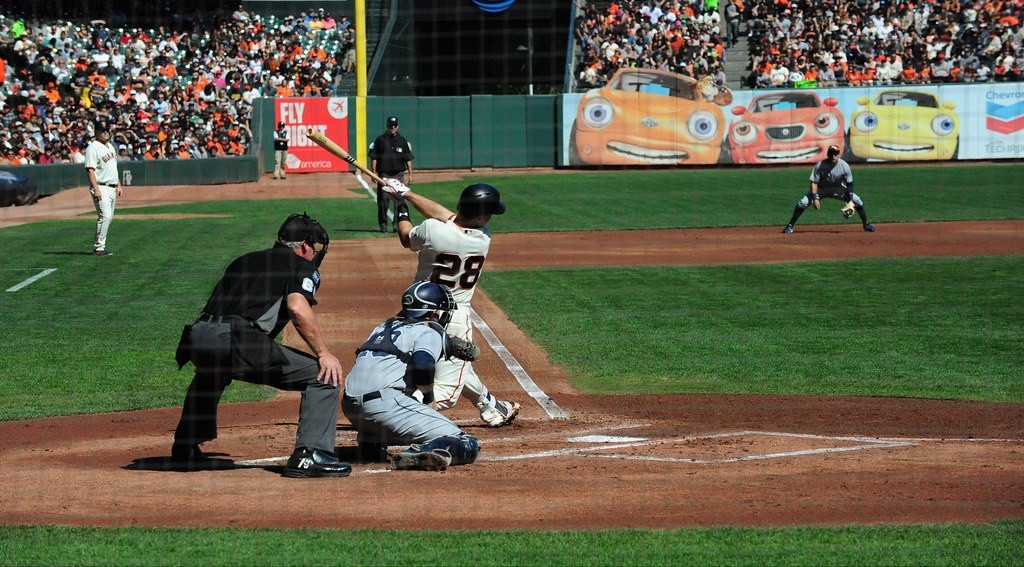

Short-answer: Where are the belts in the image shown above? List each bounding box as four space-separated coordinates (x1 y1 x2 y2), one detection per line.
99 183 116 188
199 314 232 323
343 390 381 403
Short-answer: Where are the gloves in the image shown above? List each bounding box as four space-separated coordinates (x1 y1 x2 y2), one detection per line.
381 177 410 197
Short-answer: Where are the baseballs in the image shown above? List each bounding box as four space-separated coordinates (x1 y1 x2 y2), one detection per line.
702 85 714 97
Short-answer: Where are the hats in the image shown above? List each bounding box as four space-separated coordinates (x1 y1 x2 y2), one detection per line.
95 122 110 131
388 117 398 125
278 122 286 128
828 145 839 154
278 216 325 245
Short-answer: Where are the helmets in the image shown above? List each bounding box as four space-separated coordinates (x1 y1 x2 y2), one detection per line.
395 280 457 318
457 183 506 218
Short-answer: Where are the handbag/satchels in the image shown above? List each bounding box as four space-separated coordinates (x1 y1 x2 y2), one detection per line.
231 316 290 378
175 324 193 370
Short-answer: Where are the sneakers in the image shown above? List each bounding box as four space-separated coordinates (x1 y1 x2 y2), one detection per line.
487 402 520 428
863 225 875 232
392 450 447 471
358 443 391 464
783 226 793 233
281 450 352 477
171 441 204 463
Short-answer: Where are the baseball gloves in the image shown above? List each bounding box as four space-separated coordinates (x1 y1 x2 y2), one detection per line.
841 202 855 220
448 335 480 364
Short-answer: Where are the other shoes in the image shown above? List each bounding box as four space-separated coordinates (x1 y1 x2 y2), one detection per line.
380 226 386 232
393 227 398 232
94 250 114 256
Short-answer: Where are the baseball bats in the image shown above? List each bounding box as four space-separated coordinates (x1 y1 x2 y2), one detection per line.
306 126 386 186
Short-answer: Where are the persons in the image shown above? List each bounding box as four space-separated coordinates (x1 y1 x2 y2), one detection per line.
0 6 357 165
368 117 416 233
172 213 352 478
342 281 480 470
783 145 875 234
274 122 289 179
382 177 520 427
574 0 727 88
724 0 1024 89
85 121 122 255
